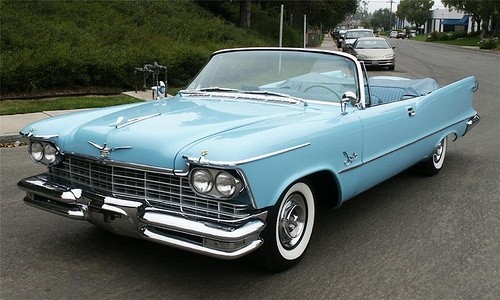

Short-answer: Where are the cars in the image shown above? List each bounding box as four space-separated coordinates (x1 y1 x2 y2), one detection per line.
331 28 379 51
348 36 396 71
396 33 405 39
389 31 397 38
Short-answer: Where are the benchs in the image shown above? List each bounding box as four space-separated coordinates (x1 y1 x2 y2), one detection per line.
261 88 381 106
293 82 408 105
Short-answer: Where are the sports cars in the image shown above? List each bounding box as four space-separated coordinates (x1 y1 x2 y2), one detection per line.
16 47 481 270
